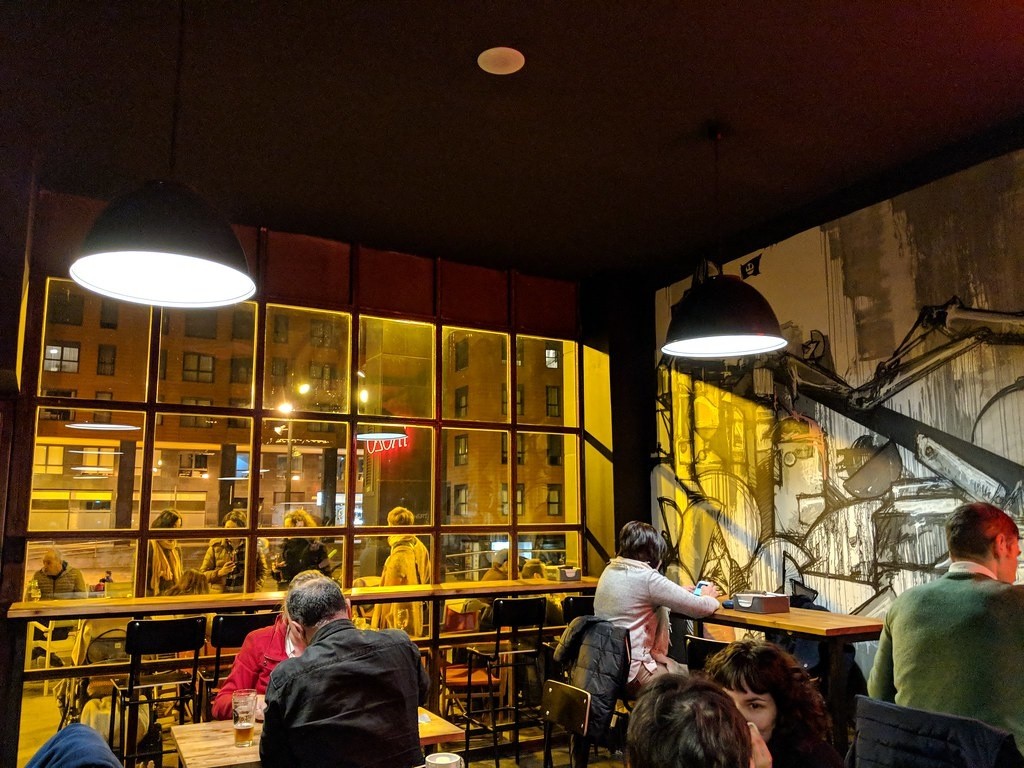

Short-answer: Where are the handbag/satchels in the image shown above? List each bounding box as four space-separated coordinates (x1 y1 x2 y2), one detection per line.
83 627 130 664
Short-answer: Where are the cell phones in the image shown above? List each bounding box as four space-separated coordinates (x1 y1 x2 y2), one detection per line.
693 581 710 597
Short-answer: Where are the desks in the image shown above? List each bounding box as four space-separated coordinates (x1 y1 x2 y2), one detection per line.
7 576 598 617
171 706 467 768
670 605 883 758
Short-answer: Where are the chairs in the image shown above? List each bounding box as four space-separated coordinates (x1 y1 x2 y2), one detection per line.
553 615 644 768
25 574 600 768
766 595 869 732
683 635 731 675
25 723 125 768
843 694 1024 768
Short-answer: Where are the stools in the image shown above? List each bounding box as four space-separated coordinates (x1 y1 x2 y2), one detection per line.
466 596 548 768
195 612 278 725
109 616 206 768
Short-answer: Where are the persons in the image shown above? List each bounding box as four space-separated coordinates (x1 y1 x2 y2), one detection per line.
593 520 721 688
370 506 431 637
212 570 329 720
200 510 267 594
480 547 564 709
131 506 184 596
867 502 1024 758
24 546 87 660
163 568 210 596
270 508 332 592
259 576 432 768
354 579 366 587
699 636 844 768
94 578 108 592
625 672 752 768
104 570 113 583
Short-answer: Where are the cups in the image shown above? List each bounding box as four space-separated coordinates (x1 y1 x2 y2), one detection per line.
232 689 257 708
26 580 39 603
233 697 255 747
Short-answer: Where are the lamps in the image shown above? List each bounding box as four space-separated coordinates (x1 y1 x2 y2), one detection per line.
356 407 407 441
66 0 257 310
660 118 789 360
